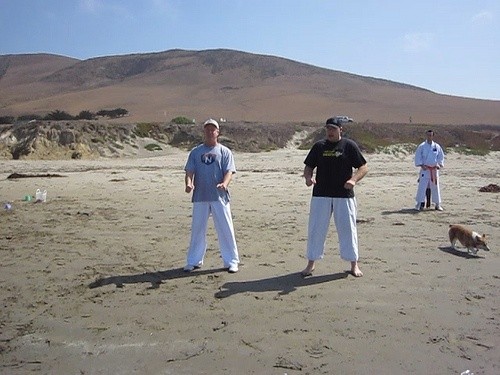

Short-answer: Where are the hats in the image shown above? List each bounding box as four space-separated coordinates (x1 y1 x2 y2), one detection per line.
203 118 219 128
324 118 341 129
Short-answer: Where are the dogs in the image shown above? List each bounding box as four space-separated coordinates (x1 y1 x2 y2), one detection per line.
449 225 490 255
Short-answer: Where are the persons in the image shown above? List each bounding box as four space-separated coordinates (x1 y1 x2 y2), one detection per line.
184 119 238 272
302 117 368 277
415 130 445 211
417 174 431 210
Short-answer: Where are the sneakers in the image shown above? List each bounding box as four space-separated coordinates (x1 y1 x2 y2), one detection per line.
184 265 194 271
229 263 238 272
